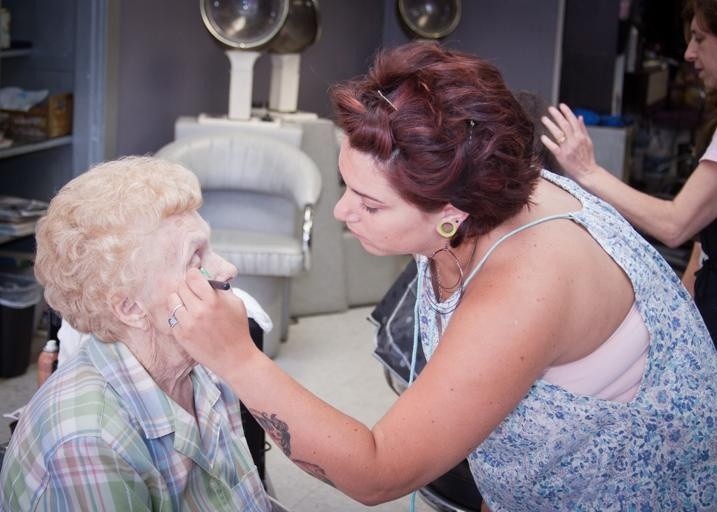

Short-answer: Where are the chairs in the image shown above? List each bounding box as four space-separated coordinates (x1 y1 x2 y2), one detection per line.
147 134 324 361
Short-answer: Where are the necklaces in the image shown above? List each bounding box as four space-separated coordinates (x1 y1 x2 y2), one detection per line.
434 237 478 301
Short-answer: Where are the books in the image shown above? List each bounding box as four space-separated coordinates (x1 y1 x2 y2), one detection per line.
0 194 51 312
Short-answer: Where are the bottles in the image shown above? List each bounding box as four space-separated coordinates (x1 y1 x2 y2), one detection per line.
39 339 57 382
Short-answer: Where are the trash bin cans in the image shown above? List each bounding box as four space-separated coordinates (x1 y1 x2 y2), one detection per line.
0 272 42 378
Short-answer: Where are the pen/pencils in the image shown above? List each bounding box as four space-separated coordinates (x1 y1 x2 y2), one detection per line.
208 280 231 290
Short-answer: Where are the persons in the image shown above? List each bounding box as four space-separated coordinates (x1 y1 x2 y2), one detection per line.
0 155 275 511
163 38 715 512
534 0 716 353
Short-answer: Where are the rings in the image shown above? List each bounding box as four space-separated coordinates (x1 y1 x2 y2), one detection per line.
170 303 186 316
555 134 567 145
168 312 178 326
